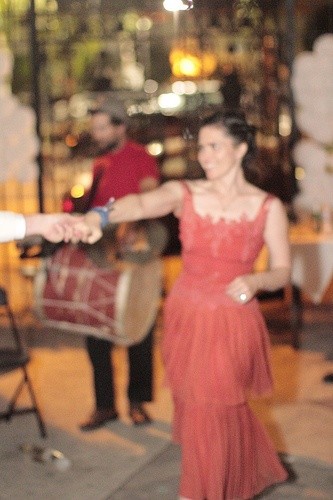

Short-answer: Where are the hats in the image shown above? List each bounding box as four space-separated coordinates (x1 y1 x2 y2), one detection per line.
88 100 132 126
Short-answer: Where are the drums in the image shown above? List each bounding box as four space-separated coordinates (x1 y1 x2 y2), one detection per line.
35 242 161 346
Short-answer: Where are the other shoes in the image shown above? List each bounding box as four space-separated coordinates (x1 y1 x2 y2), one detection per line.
129 401 149 426
80 407 118 431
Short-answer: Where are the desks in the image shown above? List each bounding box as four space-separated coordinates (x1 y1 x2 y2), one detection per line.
287 229 333 353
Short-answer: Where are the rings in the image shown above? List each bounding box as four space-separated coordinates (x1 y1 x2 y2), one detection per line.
239 293 247 301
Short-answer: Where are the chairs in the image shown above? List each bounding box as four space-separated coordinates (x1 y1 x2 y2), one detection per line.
0 286 48 438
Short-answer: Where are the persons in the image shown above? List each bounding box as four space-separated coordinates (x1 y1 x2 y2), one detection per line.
55 111 293 500
0 109 163 433
